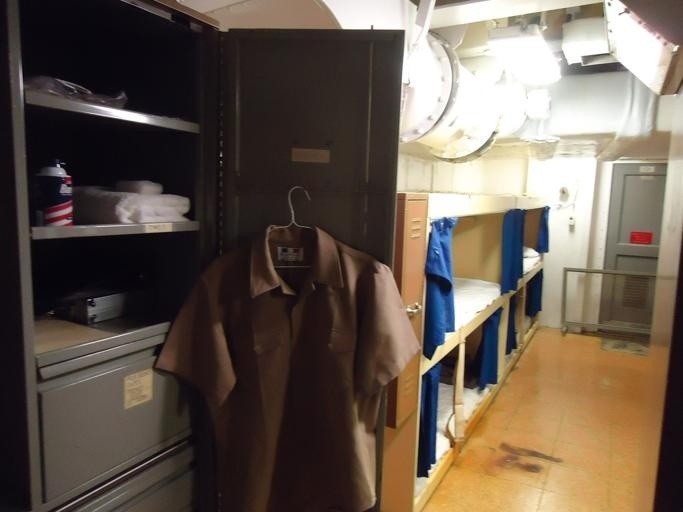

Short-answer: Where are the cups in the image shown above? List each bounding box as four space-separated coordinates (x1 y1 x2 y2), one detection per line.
32 175 74 228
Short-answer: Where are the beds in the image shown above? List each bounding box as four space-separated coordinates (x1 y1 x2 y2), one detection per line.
415 193 548 511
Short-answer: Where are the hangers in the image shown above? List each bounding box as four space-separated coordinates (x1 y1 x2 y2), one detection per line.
268 186 317 276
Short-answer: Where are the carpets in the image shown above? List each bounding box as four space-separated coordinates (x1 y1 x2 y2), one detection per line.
600 338 650 360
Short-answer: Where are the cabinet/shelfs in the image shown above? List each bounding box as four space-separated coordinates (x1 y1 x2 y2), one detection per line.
375 190 432 511
2 0 401 363
27 337 221 512
